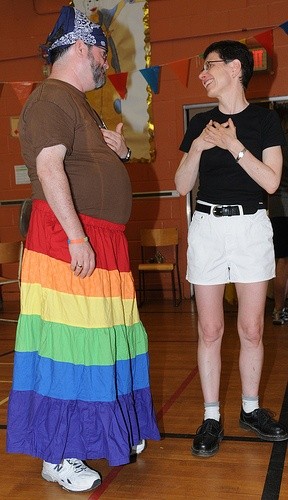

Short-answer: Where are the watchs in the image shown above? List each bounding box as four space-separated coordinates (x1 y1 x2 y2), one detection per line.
121 147 131 162
234 148 247 163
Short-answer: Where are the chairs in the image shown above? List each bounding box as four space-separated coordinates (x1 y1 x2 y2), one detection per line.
0 240 24 323
138 228 183 308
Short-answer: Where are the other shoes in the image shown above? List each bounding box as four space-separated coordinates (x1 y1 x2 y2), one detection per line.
272 308 288 325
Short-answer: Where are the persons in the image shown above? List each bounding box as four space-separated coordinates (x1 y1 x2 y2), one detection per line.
268 115 288 325
5 7 164 493
174 39 288 458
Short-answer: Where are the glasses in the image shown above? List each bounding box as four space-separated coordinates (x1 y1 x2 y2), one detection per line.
203 60 234 73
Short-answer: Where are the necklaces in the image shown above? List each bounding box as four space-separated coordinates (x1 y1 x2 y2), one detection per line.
82 99 107 129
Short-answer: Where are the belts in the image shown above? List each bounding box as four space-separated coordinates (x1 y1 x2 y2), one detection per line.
195 202 267 217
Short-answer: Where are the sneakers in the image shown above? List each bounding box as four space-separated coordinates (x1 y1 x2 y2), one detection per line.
130 437 145 455
41 457 101 491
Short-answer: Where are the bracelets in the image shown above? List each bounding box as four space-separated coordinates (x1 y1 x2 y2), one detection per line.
68 237 88 244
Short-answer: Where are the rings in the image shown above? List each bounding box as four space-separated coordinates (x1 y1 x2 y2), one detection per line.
78 265 82 267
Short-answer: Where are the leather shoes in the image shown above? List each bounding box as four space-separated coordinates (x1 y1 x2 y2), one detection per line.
191 415 225 457
239 405 288 441
